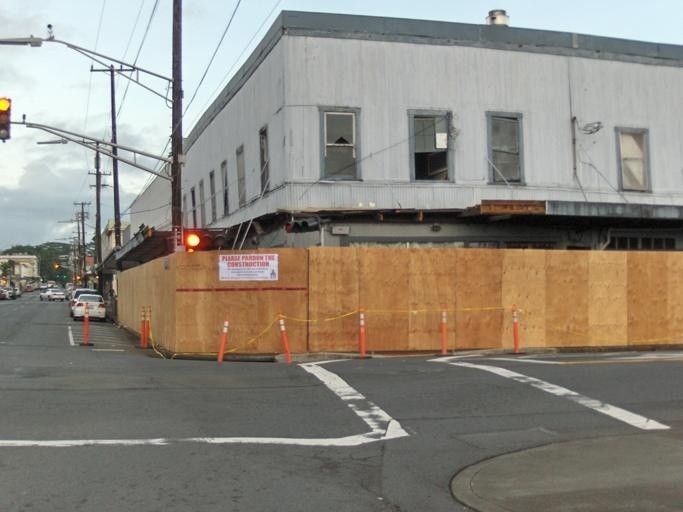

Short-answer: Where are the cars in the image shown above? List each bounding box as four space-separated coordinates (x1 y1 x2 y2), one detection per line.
0 281 55 300
39 288 65 302
64 282 106 321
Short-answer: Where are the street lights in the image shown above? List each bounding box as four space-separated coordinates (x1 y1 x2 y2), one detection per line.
0 23 182 246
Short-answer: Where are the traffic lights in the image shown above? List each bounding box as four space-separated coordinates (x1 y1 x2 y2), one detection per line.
0 97 12 141
184 227 225 247
